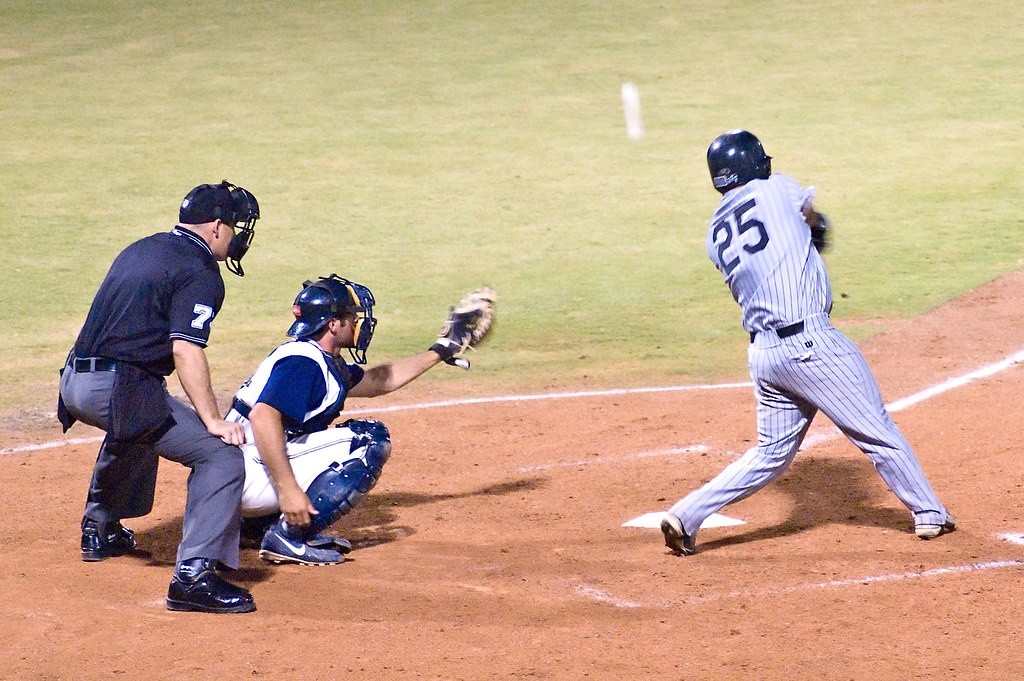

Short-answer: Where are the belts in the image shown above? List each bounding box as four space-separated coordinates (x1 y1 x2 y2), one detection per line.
750 321 806 343
233 397 253 420
69 354 117 373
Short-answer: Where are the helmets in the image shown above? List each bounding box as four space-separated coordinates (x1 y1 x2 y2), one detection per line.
179 184 238 225
707 130 772 194
285 280 354 338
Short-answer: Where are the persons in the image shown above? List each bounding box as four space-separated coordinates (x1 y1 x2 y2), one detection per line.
661 131 955 555
226 274 495 565
58 180 261 614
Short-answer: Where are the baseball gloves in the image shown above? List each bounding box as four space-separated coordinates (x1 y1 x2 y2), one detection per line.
429 285 497 370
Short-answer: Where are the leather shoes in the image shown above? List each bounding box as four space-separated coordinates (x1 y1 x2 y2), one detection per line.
165 575 255 612
81 521 135 561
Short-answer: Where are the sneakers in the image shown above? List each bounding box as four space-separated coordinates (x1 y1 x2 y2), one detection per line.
661 514 696 555
915 509 955 539
258 526 344 566
268 521 350 555
